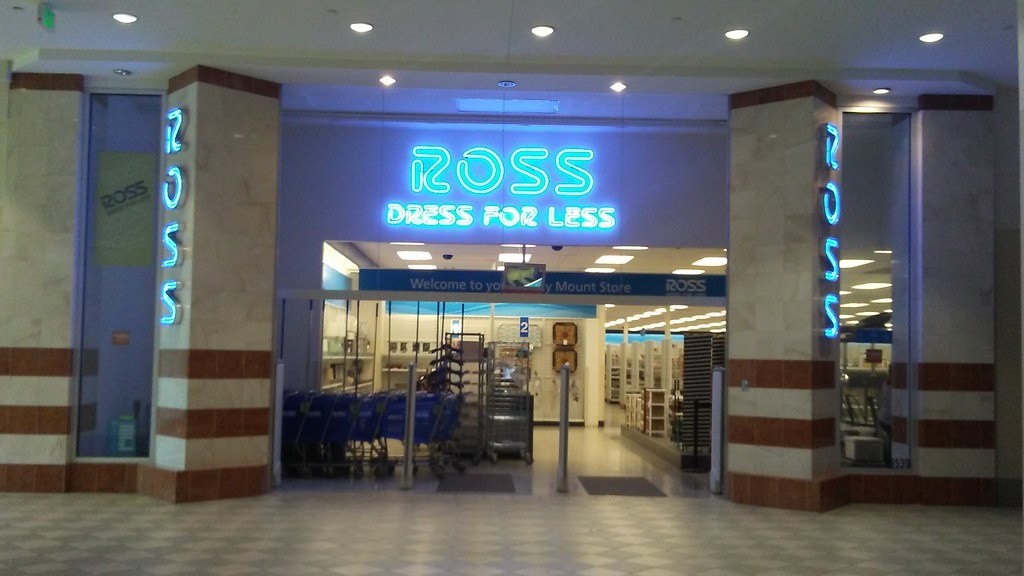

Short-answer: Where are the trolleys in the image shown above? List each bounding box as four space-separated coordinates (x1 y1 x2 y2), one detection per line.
284 388 474 481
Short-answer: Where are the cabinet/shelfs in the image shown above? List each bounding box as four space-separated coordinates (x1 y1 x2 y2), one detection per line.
320 335 374 396
381 339 441 394
606 339 686 452
